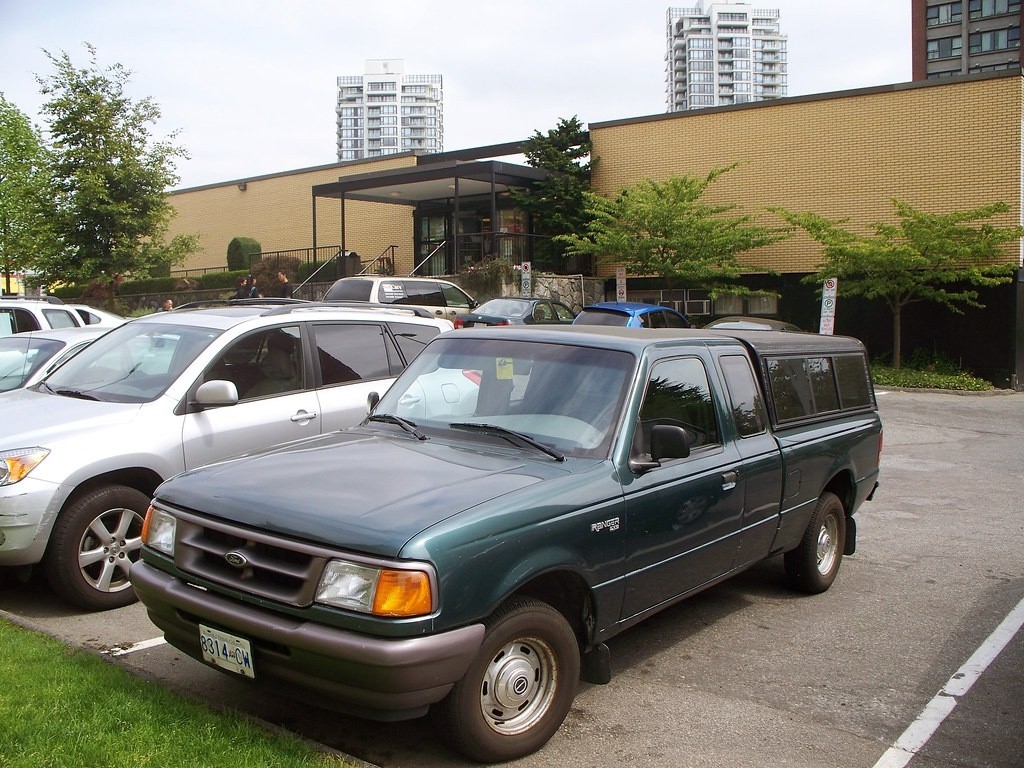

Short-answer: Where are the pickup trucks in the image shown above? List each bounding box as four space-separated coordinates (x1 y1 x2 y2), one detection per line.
126 321 883 768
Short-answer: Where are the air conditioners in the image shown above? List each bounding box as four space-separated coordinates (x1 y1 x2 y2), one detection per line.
685 300 711 315
658 301 682 314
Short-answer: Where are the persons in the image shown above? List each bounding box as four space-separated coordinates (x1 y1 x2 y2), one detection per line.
158 300 174 313
278 270 293 298
235 275 268 298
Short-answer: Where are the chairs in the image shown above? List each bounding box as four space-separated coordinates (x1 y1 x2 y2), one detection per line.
243 334 294 399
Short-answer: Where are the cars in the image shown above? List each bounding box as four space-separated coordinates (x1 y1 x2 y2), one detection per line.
701 315 803 331
0 291 183 390
570 302 696 329
452 296 576 334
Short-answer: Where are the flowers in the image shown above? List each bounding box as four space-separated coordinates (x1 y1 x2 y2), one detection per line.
458 252 521 294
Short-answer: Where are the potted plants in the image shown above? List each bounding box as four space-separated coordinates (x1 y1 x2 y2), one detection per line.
709 287 780 316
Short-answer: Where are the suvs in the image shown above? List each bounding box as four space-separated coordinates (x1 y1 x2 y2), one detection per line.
323 273 481 323
2 294 462 615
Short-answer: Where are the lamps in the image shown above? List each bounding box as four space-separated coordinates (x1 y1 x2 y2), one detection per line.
238 182 246 191
